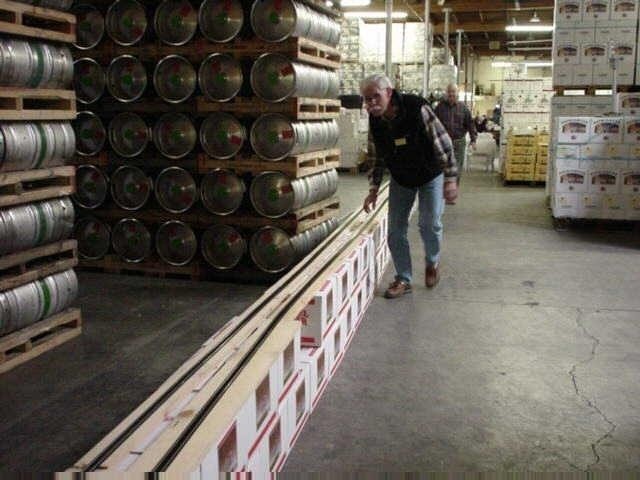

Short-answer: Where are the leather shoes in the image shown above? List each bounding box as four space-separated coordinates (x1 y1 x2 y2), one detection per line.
424 265 440 288
384 277 413 298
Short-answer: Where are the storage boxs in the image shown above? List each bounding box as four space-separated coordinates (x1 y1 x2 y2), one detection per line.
497 1 639 223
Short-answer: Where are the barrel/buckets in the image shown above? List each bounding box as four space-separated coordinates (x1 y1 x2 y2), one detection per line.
2 35 79 336
26 2 339 274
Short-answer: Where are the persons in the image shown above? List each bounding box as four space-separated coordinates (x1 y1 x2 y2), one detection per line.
434 81 478 205
360 74 457 299
479 115 487 131
